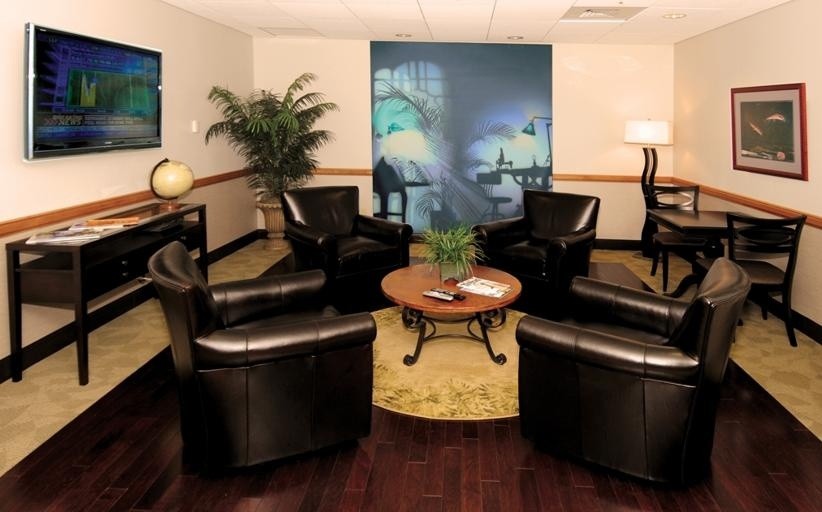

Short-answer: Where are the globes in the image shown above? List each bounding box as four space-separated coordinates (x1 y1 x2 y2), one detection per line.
150 158 194 209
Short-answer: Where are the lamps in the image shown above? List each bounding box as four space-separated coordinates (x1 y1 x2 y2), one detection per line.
522 116 552 168
623 119 673 258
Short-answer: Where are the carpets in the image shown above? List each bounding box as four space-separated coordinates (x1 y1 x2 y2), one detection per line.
370 305 530 422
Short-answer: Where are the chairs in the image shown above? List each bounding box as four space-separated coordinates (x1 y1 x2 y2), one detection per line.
515 257 752 490
645 185 725 291
281 186 414 315
475 171 512 222
693 210 806 347
147 241 376 479
471 190 600 323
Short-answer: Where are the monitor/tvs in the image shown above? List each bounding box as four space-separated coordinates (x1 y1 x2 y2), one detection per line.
25 22 163 161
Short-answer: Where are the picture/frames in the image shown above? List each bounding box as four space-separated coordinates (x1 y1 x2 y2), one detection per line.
731 83 809 180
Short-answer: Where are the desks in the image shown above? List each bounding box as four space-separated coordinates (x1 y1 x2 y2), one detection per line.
6 202 208 386
497 168 552 191
646 208 757 326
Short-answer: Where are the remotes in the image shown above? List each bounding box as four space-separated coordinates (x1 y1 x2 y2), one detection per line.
430 288 465 301
422 290 454 302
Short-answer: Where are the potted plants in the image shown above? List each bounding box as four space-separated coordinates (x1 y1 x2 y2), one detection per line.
420 223 490 284
205 74 338 251
374 82 517 232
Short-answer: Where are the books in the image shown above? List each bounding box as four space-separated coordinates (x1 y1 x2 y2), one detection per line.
456 277 511 298
25 216 140 245
